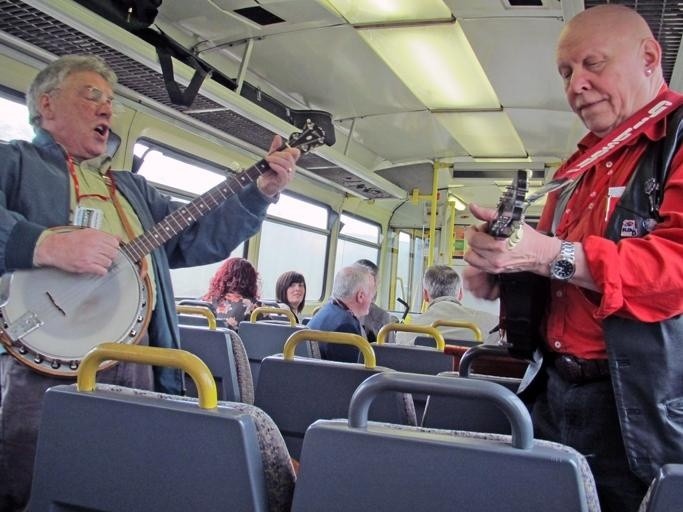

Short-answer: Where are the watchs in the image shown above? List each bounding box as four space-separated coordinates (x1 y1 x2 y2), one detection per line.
546 236 578 283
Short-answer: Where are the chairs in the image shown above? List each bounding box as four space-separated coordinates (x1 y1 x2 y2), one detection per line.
21 342 268 510
173 298 549 460
638 463 682 512
289 369 604 512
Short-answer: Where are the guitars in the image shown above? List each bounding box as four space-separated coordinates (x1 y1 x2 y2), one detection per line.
484 170 552 352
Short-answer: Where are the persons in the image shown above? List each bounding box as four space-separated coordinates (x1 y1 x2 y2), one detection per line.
305 262 378 371
389 261 510 357
198 254 272 335
349 256 402 343
454 2 682 512
0 50 301 511
273 266 308 331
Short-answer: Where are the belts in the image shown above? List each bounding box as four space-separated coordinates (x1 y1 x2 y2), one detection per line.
539 343 611 391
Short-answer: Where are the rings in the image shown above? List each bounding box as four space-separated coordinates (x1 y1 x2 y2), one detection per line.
286 167 294 175
